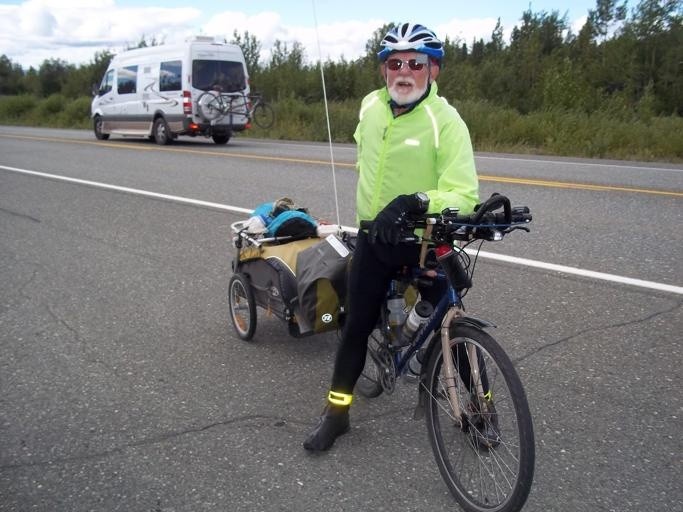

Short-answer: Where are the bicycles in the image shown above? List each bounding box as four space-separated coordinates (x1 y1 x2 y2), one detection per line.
338 191 536 512
196 85 275 129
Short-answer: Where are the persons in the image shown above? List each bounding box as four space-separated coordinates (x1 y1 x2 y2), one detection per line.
300 21 503 452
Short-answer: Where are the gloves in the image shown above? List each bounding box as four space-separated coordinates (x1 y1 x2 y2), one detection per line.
366 193 421 246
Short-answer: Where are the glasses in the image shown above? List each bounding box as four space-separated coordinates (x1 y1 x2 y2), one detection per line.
385 58 428 71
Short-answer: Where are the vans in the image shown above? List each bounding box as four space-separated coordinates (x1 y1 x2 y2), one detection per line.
89 41 253 146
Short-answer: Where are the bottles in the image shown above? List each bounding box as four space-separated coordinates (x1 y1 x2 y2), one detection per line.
387 288 429 342
406 348 425 377
434 245 470 288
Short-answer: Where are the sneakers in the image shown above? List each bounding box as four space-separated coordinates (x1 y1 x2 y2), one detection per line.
474 402 501 449
302 402 352 452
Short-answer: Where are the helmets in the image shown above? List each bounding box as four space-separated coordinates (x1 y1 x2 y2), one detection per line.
376 22 445 64
